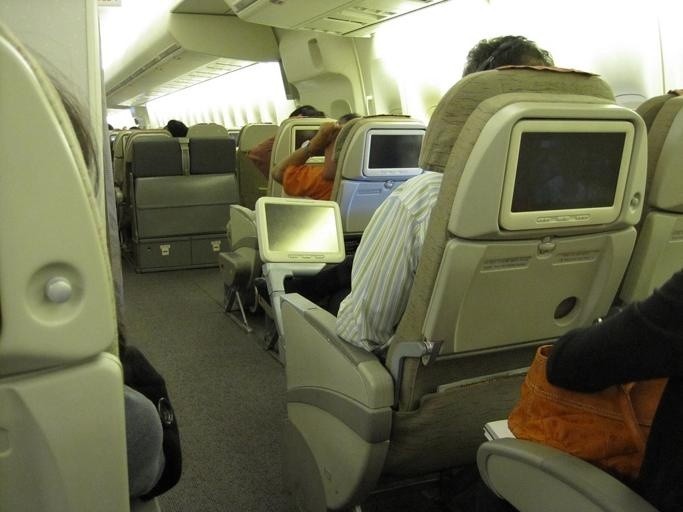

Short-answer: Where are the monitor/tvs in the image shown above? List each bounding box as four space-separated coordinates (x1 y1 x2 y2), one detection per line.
499 121 635 231
362 129 425 178
292 126 325 164
255 197 346 262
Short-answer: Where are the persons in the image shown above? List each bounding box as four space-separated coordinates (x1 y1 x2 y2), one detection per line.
336 262 681 509
40 72 182 511
293 34 556 355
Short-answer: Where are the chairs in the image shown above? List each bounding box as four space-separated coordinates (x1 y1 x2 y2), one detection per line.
104 106 427 273
218 66 682 511
1 38 129 511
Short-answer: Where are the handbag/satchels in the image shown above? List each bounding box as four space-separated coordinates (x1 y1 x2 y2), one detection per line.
508 346 670 484
